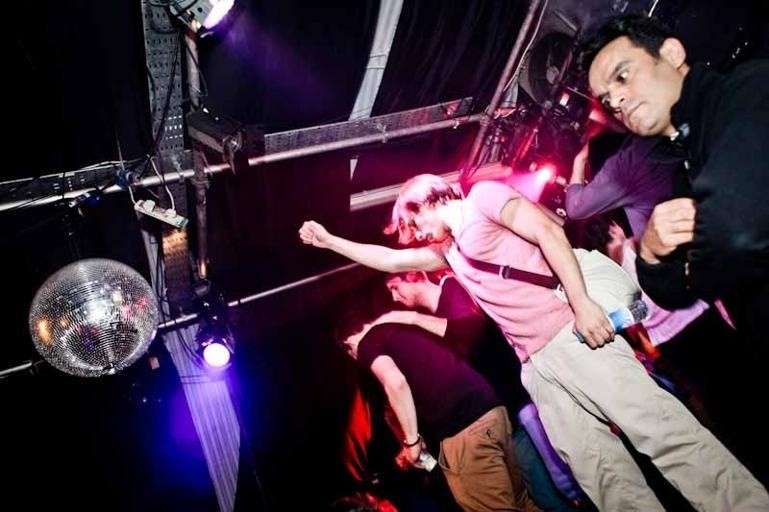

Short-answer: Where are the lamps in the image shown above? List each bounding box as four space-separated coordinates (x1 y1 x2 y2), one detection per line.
176 2 232 33
186 109 248 161
193 289 237 373
492 74 622 222
27 197 159 379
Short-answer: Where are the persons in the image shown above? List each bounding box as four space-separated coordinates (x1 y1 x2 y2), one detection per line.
295 7 768 511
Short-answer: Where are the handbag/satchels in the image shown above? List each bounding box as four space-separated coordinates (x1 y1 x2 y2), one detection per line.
557 246 651 344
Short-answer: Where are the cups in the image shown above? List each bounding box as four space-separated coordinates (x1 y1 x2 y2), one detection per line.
415 449 439 473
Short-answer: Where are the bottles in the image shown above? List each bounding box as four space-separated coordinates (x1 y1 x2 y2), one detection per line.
576 299 652 344
